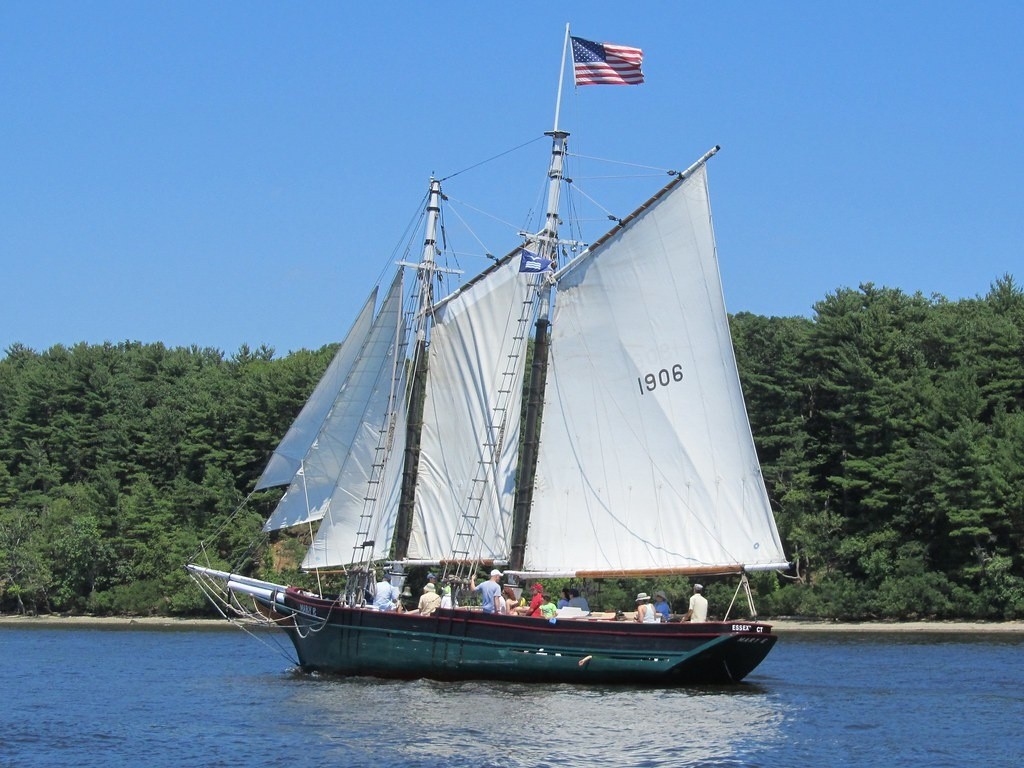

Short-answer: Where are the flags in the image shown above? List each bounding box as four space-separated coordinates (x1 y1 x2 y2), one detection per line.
518 249 552 273
570 34 645 87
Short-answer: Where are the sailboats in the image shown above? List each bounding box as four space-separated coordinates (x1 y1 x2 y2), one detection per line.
183 131 794 689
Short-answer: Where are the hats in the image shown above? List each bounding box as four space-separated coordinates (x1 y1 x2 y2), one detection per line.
383 568 703 602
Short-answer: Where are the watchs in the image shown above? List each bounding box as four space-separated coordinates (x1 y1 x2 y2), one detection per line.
497 610 500 612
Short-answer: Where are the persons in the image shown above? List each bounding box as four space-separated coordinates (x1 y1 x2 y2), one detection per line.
654 591 670 622
539 594 558 620
683 584 708 622
373 574 402 613
568 589 590 611
524 584 544 618
503 588 519 615
634 593 656 622
427 573 437 583
418 583 442 616
471 570 503 615
557 589 570 608
